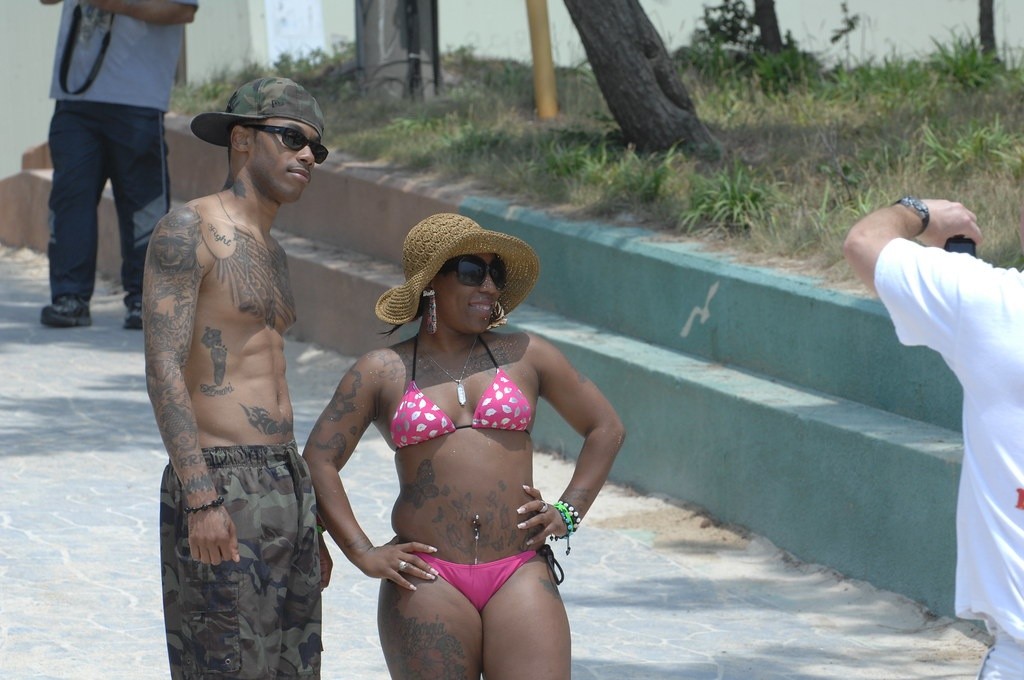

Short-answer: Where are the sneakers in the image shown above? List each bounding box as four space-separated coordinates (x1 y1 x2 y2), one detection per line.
122 294 144 329
40 294 92 326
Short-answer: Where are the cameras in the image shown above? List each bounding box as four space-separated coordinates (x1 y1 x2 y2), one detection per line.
944 238 977 259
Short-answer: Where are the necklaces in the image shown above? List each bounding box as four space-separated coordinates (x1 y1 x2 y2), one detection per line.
416 334 476 406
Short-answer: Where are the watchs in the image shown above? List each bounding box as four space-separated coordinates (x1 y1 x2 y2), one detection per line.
897 197 929 236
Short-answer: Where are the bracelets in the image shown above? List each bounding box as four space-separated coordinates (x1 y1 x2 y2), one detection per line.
185 496 225 513
550 501 581 556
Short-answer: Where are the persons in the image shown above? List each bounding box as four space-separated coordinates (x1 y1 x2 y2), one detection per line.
845 198 1024 680
42 1 196 332
303 213 626 680
143 77 333 680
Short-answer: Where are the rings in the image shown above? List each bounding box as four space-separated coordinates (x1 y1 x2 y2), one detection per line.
538 500 547 513
399 562 406 571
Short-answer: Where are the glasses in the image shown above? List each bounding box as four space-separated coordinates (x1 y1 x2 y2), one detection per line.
240 123 329 164
447 254 506 291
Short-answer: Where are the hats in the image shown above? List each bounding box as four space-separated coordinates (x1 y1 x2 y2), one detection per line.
189 76 324 146
374 213 540 324
